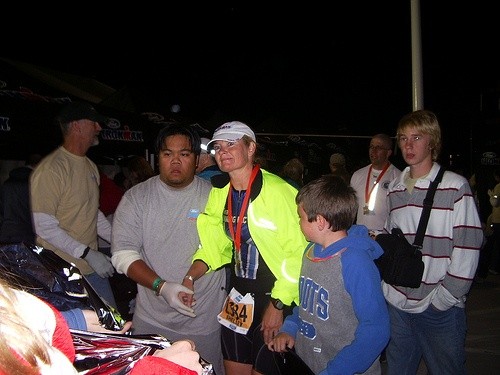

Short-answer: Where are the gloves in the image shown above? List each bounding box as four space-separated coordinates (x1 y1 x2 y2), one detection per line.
158 282 197 318
80 246 114 279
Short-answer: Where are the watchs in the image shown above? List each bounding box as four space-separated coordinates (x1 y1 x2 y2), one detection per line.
269 297 285 310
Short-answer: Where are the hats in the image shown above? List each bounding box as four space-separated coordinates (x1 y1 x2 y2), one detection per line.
56 102 109 124
329 153 345 168
207 120 256 150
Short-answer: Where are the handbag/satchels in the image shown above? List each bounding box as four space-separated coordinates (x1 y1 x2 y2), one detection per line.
375 228 425 289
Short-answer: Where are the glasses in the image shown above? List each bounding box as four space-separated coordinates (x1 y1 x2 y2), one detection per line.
368 145 391 152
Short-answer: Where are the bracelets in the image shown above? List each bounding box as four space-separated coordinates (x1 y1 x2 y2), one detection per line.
185 274 196 284
151 277 165 295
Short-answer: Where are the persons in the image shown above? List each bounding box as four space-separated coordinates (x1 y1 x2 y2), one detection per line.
282 158 303 189
61 308 132 334
0 106 154 320
0 278 203 375
329 153 348 175
179 121 309 375
468 168 500 278
110 123 227 375
350 134 402 363
194 138 222 180
381 112 483 375
267 175 391 375
